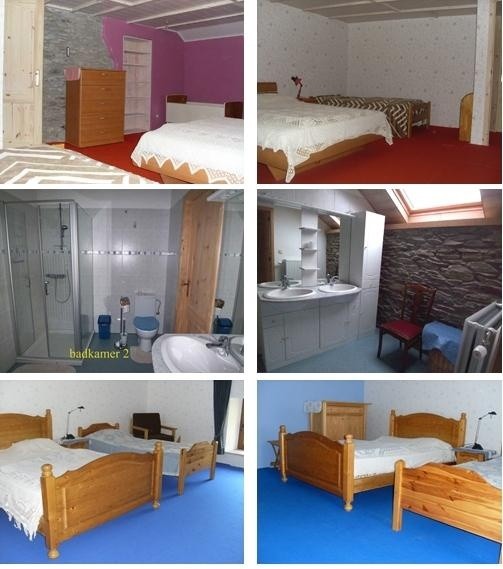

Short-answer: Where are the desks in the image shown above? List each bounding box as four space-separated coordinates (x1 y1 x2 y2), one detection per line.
422 320 463 372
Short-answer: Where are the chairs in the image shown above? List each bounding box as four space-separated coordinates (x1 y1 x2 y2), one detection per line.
130 412 180 442
376 282 437 372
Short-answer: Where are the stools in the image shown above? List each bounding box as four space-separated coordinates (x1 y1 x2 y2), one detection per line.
268 440 279 471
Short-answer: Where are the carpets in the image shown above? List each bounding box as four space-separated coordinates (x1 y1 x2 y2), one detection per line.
12 363 76 373
129 345 153 364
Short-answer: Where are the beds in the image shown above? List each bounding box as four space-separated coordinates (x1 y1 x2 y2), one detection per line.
392 455 503 564
279 409 466 511
78 423 218 495
130 116 244 184
165 94 243 123
307 92 431 138
257 81 394 183
0 409 164 560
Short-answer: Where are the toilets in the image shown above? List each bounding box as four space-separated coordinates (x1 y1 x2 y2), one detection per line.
133 292 159 351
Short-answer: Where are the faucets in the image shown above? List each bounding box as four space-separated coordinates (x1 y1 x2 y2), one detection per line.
205 336 229 357
327 274 331 283
283 276 291 290
330 276 337 285
279 279 284 288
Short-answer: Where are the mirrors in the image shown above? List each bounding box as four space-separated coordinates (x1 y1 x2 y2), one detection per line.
257 197 352 288
207 189 244 366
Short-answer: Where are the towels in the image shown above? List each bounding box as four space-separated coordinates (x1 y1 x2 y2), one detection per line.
64 66 81 81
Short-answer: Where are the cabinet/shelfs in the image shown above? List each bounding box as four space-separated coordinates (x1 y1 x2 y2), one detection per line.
65 68 126 148
258 290 362 372
298 227 322 270
310 401 372 438
458 93 474 142
123 34 154 135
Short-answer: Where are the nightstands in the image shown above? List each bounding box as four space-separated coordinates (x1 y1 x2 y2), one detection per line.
53 436 89 448
455 445 497 465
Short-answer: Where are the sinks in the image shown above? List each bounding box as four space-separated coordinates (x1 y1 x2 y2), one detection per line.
317 279 335 284
161 336 243 373
319 284 357 293
259 281 300 288
262 288 315 300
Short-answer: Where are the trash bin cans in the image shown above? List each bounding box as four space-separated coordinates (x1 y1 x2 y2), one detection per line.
98 315 111 338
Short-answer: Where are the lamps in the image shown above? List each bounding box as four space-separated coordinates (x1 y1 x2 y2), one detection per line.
472 411 497 451
63 406 84 439
292 76 306 99
